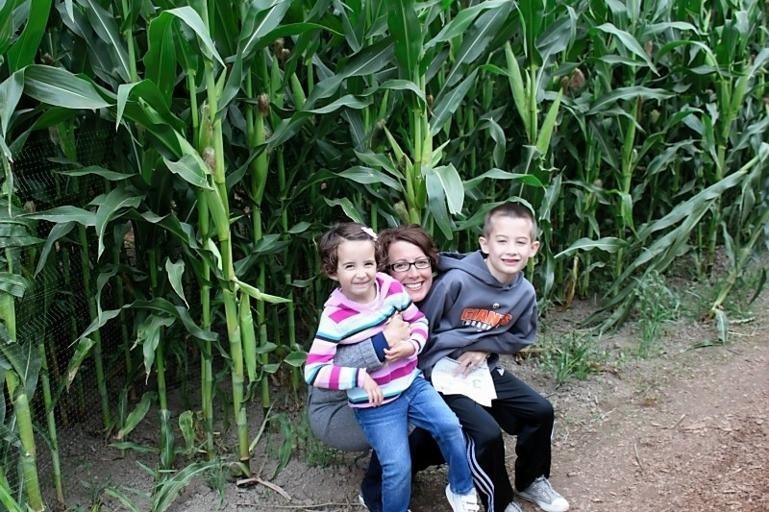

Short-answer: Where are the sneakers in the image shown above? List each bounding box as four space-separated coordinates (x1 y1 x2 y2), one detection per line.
503 501 521 511
512 473 571 511
358 494 368 509
444 482 481 512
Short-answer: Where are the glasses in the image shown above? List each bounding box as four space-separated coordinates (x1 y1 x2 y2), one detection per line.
384 254 433 274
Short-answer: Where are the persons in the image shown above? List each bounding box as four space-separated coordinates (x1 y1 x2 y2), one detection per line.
418 202 569 512
305 223 446 512
304 223 481 511
